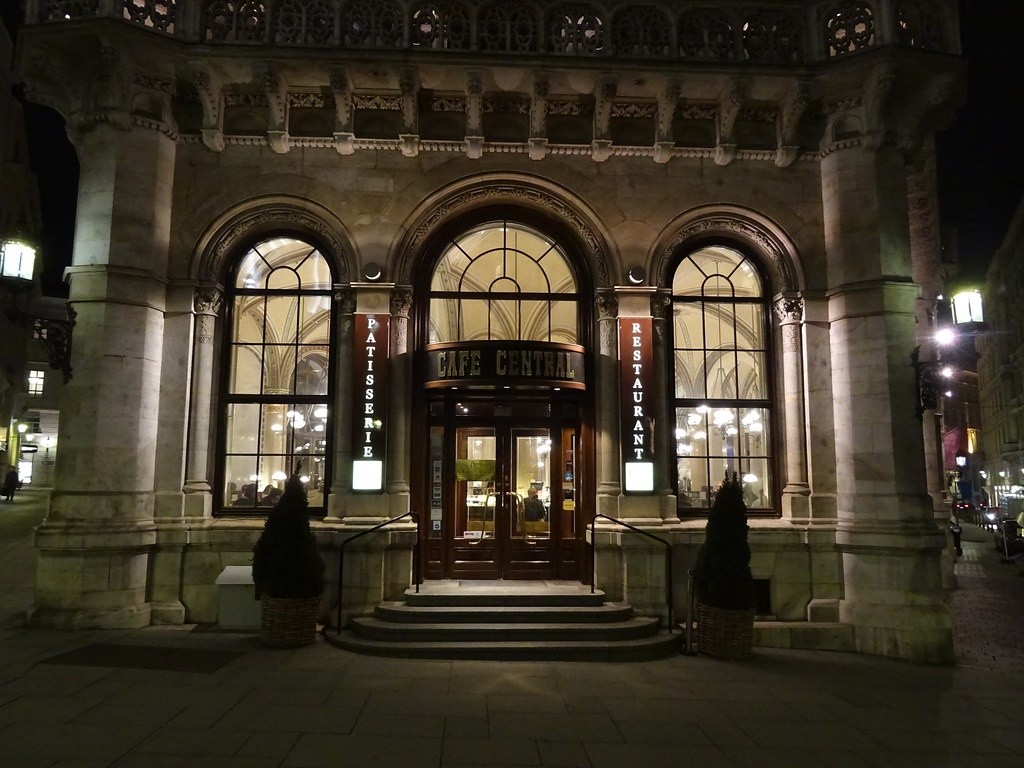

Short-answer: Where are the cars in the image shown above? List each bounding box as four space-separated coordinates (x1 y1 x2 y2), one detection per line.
994 520 1024 555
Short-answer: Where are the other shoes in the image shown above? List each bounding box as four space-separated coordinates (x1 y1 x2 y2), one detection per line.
6 500 13 503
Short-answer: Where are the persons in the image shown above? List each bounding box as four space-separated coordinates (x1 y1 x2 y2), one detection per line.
5 467 19 504
523 487 546 523
230 482 283 505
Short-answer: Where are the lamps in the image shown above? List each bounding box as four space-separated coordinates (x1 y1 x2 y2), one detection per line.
0 229 78 385
272 272 331 435
911 270 988 425
675 261 762 439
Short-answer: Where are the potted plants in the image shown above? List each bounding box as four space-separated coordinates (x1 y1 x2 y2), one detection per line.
252 474 325 648
691 469 755 662
